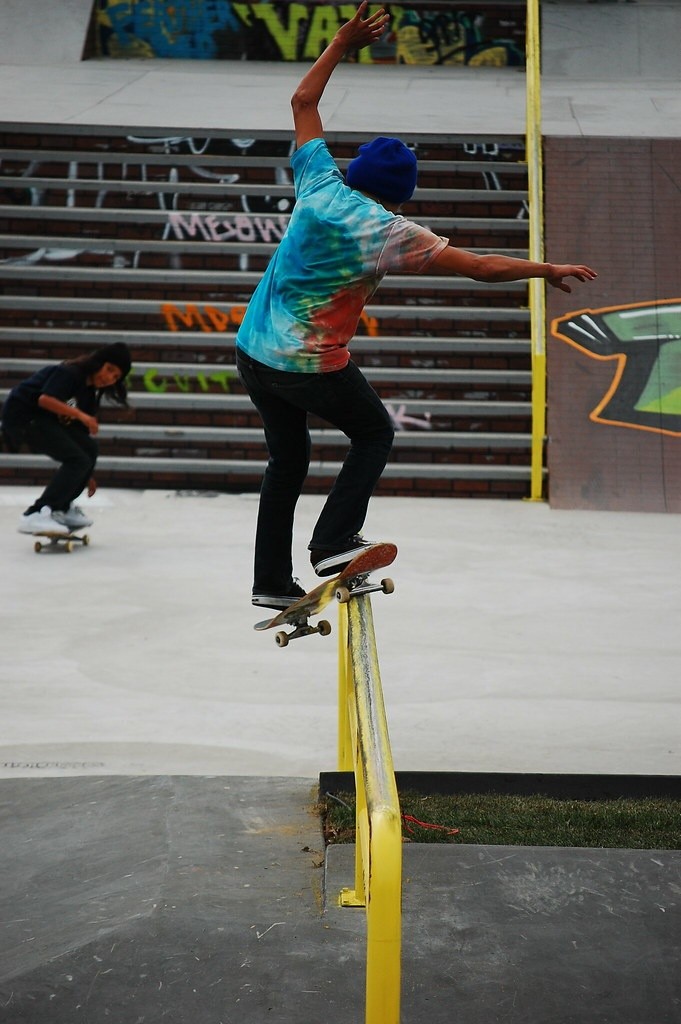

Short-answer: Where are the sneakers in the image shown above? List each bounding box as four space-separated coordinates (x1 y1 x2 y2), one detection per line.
17 505 70 535
252 582 307 610
310 532 375 577
52 501 93 526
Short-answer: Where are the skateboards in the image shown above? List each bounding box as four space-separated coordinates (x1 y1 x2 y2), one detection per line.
253 541 398 648
31 508 90 554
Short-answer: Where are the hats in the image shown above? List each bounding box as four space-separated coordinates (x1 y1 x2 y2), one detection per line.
346 137 417 202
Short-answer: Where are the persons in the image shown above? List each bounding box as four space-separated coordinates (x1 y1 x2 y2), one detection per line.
0 341 132 536
236 1 597 611
